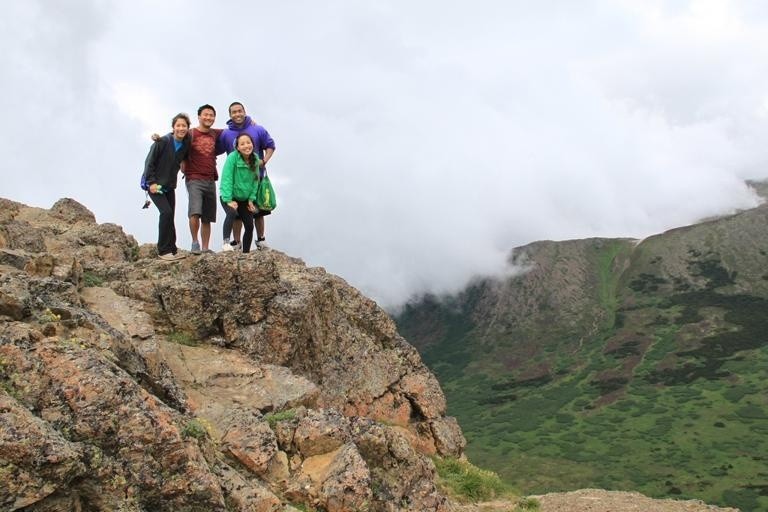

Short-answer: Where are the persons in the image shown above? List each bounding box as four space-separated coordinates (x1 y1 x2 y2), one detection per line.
219 134 259 254
141 113 194 262
217 102 276 251
152 104 255 254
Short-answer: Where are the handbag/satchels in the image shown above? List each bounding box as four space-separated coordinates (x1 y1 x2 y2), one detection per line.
141 174 148 191
257 177 276 212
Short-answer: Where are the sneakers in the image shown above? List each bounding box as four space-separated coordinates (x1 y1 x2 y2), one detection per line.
192 242 201 255
203 249 215 253
173 252 186 259
157 253 174 260
230 240 242 251
222 242 233 251
255 240 272 252
